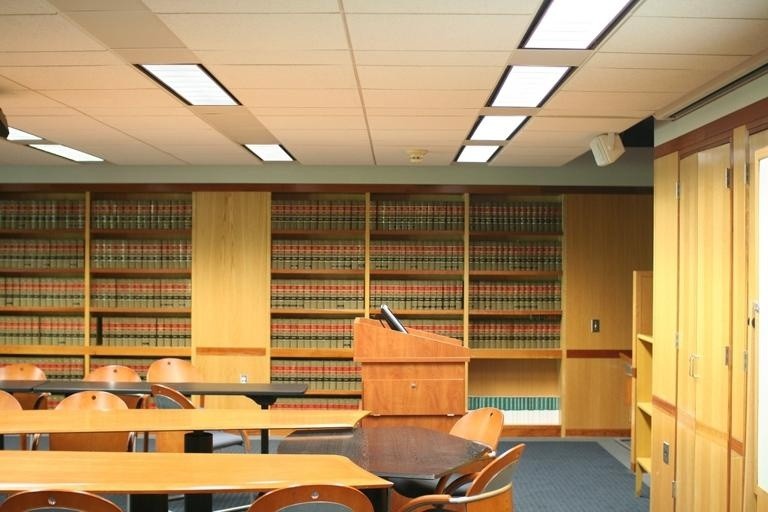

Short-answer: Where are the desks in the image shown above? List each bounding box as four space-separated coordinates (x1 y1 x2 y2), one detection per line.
277 426 491 480
1 380 308 453
1 451 393 511
1 410 397 511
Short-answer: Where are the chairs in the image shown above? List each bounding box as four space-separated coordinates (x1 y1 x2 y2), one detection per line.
151 384 249 447
1 490 121 511
248 484 375 511
147 359 205 408
0 390 24 410
32 391 135 451
399 442 525 511
1 364 47 408
434 407 504 493
83 365 141 381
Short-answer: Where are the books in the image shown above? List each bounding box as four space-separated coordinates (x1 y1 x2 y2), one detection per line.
369 201 463 345
88 197 191 380
469 396 560 426
468 201 561 348
272 196 365 408
1 196 85 380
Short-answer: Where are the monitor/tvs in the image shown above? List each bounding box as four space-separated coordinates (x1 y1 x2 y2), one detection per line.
378 302 406 333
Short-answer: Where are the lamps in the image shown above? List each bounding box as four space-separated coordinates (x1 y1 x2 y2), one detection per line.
589 133 627 168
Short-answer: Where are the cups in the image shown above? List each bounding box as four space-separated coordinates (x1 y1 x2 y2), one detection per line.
240 371 248 384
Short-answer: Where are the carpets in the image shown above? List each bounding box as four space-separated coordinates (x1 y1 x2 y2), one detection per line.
1 435 652 511
614 434 632 454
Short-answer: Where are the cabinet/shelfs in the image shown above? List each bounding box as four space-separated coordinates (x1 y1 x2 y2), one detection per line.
366 184 465 352
86 183 192 380
651 126 748 512
0 183 86 379
466 185 565 441
270 183 366 409
632 269 651 496
